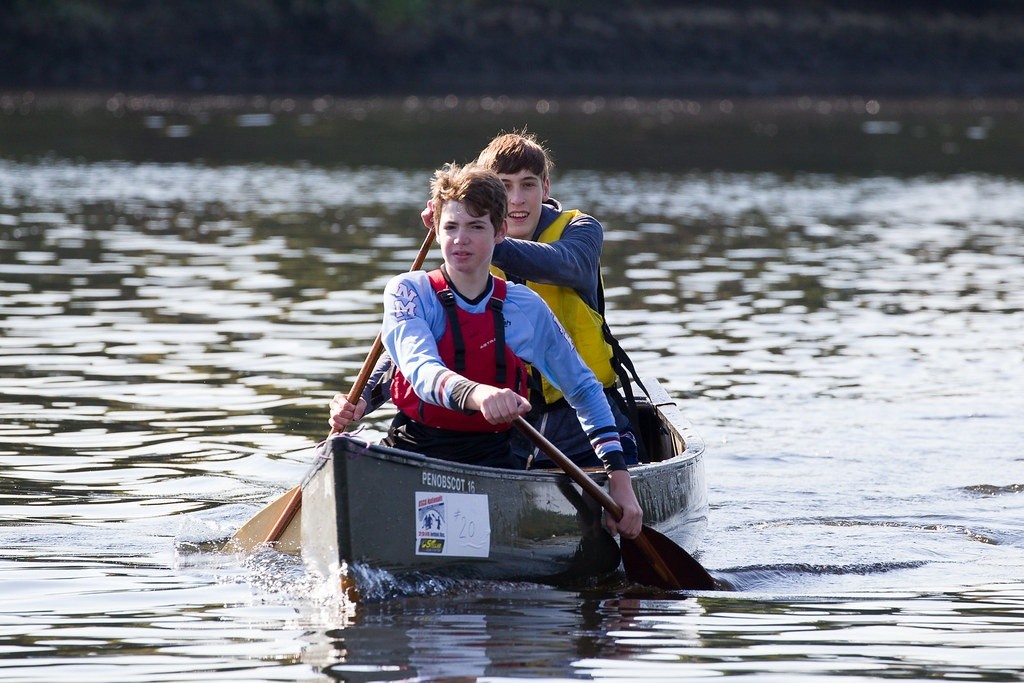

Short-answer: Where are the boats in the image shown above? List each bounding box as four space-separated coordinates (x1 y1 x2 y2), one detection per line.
295 374 700 598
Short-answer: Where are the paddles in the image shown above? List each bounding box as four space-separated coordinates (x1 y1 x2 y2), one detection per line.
217 223 438 558
511 409 712 597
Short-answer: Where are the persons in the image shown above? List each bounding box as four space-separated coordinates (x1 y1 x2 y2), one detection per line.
328 135 637 467
384 167 643 538
